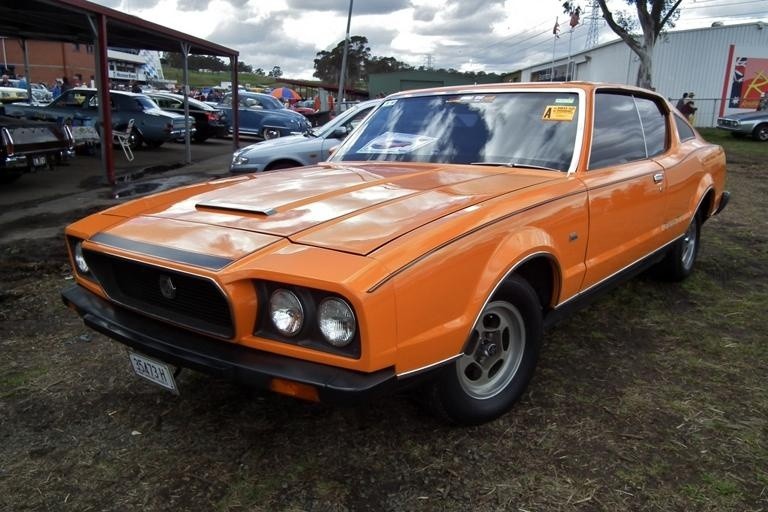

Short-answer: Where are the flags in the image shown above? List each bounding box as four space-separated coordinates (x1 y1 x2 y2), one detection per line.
568 8 580 28
552 16 560 40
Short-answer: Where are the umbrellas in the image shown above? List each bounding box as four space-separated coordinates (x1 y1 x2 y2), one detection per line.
270 86 301 105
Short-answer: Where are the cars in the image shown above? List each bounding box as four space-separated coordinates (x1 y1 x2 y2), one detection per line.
717 109 767 143
228 97 386 177
57 80 733 419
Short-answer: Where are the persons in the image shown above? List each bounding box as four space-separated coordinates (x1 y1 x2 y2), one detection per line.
675 92 687 112
679 90 697 127
281 97 346 111
763 91 768 111
0 73 14 88
50 74 142 101
15 73 26 89
170 85 226 103
756 92 765 111
